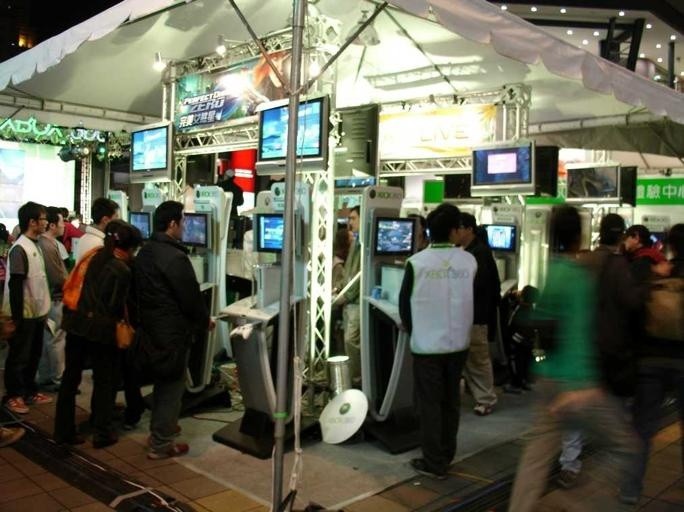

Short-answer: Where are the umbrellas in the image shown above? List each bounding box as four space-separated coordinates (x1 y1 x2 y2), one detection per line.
0 0 684 512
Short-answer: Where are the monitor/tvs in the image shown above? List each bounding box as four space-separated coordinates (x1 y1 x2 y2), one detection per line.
536 146 560 197
621 166 638 208
129 121 173 184
480 224 519 253
565 162 621 208
332 103 382 179
252 210 302 256
334 179 379 192
380 160 406 197
179 212 212 250
128 211 155 243
255 94 331 176
443 174 483 202
469 139 536 197
370 216 417 258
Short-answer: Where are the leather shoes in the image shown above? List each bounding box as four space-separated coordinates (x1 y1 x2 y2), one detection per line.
513 377 535 392
55 434 85 445
93 433 118 447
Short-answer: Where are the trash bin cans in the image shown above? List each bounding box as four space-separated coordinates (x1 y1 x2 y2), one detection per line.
325 355 353 401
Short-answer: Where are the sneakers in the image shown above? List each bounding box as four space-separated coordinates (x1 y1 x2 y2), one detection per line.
4 393 52 413
410 459 450 479
121 420 134 429
39 380 60 394
473 404 494 415
0 426 25 447
147 425 188 460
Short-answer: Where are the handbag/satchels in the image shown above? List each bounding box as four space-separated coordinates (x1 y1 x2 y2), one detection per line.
162 332 201 367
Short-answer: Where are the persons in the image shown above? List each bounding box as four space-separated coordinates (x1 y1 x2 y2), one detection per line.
1 198 215 459
218 169 243 221
333 206 361 386
229 218 253 299
530 203 684 501
399 202 501 479
508 387 644 510
